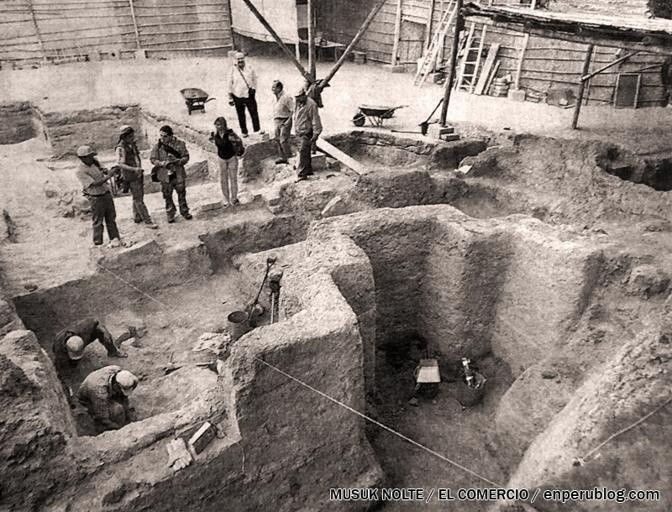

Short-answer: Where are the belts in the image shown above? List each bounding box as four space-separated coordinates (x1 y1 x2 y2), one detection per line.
85 192 112 198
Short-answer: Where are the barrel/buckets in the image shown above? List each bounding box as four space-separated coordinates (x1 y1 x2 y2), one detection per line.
490 78 509 97
457 369 486 407
354 51 367 64
228 309 249 340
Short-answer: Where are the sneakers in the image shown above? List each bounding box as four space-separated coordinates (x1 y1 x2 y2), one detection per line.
274 156 288 165
294 174 308 184
143 221 160 230
223 200 232 209
166 213 176 224
180 210 193 220
305 171 314 176
231 198 242 207
107 348 129 358
242 132 249 139
254 129 266 135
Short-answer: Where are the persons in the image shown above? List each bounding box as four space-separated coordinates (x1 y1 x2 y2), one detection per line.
209 117 241 207
227 52 261 135
77 365 139 430
151 126 193 221
115 124 156 229
270 80 294 165
53 319 127 397
293 87 323 176
76 145 122 248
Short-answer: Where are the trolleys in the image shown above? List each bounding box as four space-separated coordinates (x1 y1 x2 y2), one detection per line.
413 358 442 401
351 105 409 129
181 88 216 115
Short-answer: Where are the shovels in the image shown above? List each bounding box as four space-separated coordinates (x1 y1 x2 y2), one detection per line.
420 97 445 137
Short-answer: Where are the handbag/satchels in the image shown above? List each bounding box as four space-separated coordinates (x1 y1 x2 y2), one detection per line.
228 130 246 158
150 164 163 184
114 174 132 194
246 86 258 100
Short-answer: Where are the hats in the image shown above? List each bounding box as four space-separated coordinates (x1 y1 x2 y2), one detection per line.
76 144 98 158
118 124 136 139
65 335 87 362
115 369 136 397
292 86 307 98
